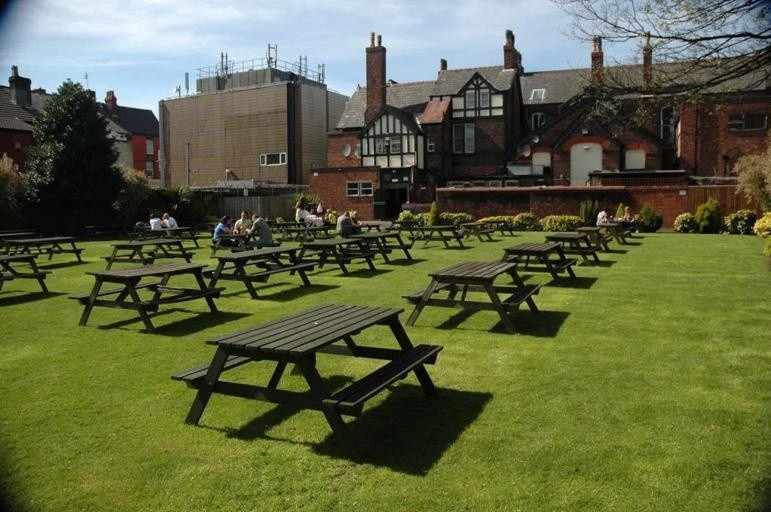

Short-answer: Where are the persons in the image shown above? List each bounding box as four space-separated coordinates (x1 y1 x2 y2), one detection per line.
233 210 252 238
293 201 360 238
163 212 179 237
623 206 636 229
246 214 273 249
213 216 237 247
150 213 163 231
596 207 614 232
